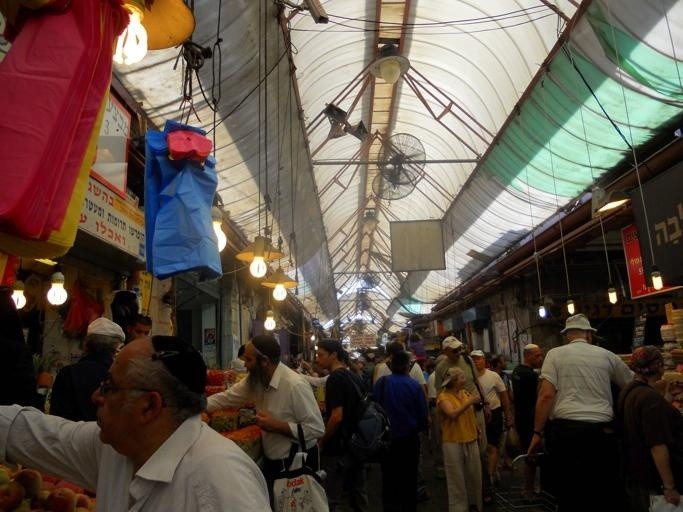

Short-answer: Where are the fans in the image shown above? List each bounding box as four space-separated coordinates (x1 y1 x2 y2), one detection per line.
349 316 367 334
358 270 381 290
374 127 427 185
370 168 418 202
353 289 371 311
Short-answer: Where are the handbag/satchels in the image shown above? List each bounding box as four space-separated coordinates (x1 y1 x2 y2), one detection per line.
650 494 683 512
270 423 330 511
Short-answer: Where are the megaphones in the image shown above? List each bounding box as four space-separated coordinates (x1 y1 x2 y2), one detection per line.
345 122 367 140
323 103 347 122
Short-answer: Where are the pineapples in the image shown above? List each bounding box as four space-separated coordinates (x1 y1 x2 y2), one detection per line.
38 356 53 386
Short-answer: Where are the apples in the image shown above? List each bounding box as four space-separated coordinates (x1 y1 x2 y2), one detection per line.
0 465 90 512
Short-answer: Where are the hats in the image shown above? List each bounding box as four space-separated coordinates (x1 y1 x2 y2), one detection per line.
559 313 597 334
471 349 485 359
251 335 282 359
442 336 463 351
439 366 465 387
86 318 124 341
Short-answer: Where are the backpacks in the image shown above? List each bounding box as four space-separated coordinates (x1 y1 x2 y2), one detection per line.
333 370 392 456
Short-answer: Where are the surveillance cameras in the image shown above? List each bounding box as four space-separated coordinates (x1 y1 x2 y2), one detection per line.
300 0 329 24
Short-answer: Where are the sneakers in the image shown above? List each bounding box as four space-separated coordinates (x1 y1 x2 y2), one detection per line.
349 494 369 510
418 488 432 500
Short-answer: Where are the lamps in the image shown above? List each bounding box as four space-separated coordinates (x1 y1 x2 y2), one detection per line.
595 190 630 213
360 208 381 232
543 55 575 313
10 265 30 310
610 15 665 291
111 0 198 70
41 263 77 309
359 34 417 90
210 21 301 331
514 71 547 321
565 28 618 305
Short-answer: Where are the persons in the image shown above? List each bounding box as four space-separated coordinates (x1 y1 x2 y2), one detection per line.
124 314 155 343
0 334 274 512
226 343 256 371
614 342 681 512
205 329 324 498
47 318 126 421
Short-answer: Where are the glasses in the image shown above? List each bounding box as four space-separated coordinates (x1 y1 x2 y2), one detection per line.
448 348 461 353
98 377 151 398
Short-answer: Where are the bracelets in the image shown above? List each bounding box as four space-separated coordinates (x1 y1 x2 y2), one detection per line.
659 484 676 491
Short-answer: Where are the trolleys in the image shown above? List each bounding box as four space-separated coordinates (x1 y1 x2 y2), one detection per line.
491 453 558 512
